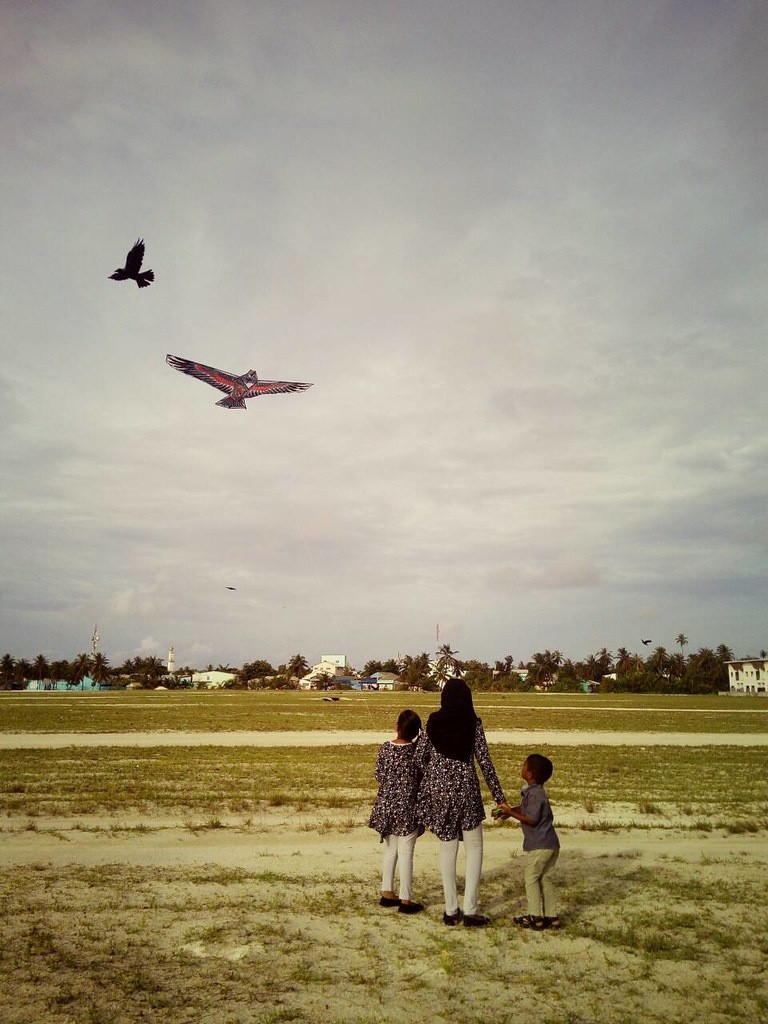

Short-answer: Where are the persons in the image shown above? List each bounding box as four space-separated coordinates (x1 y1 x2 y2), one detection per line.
419 677 509 927
491 754 564 930
368 710 430 914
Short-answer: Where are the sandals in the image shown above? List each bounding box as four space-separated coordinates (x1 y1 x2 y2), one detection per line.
513 914 562 931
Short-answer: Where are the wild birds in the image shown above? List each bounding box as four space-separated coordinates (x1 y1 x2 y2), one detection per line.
225 587 236 590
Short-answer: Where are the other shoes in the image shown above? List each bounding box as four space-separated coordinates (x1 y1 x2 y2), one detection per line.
379 897 401 907
463 915 491 927
442 908 464 926
398 901 425 914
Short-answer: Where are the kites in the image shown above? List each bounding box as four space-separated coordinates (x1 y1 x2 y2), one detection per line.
107 238 155 287
166 353 316 409
